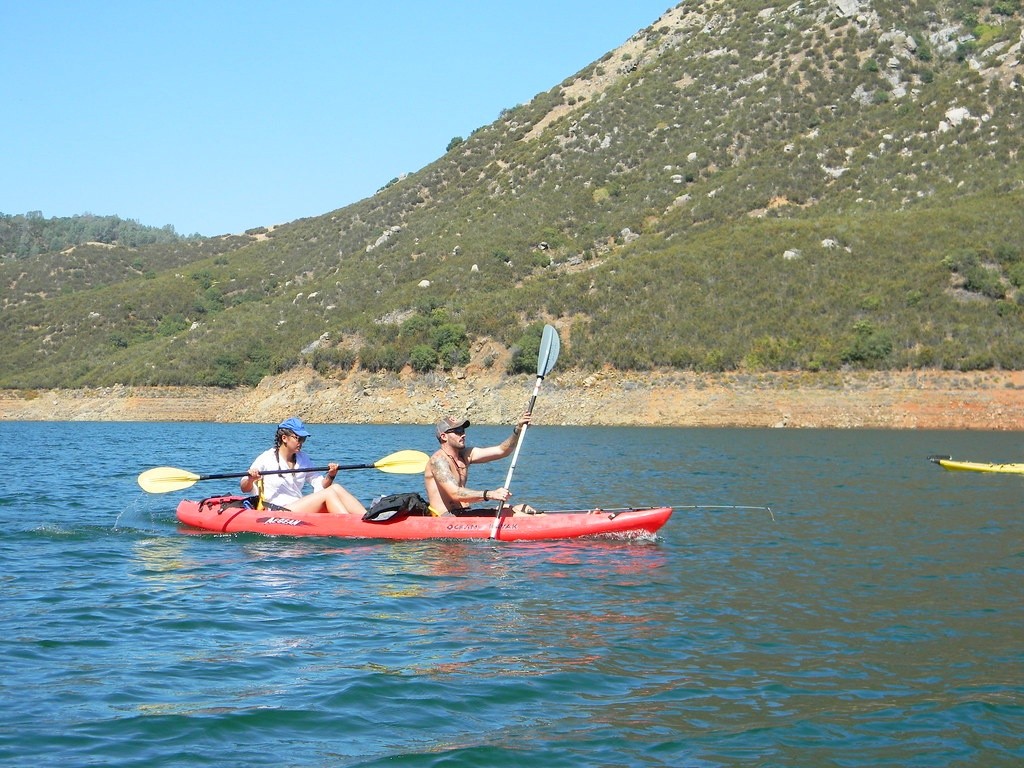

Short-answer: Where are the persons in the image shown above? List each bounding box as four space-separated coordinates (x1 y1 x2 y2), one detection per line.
423 419 592 517
240 417 367 515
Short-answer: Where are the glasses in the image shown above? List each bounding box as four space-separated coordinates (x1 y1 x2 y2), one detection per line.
289 435 306 442
445 428 465 435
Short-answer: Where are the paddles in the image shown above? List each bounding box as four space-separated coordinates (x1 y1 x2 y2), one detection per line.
137 451 430 494
488 325 560 541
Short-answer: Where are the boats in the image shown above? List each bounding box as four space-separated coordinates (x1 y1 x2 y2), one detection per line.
173 492 675 543
928 452 1024 477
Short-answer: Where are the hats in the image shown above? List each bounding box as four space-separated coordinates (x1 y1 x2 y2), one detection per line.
436 417 470 439
279 418 311 438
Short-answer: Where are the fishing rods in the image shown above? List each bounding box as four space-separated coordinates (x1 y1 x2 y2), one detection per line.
528 505 774 521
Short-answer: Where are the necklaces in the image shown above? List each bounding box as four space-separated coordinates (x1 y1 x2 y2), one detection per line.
440 446 467 468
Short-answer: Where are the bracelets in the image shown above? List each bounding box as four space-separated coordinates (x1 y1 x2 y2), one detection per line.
484 490 489 501
513 428 518 434
325 472 335 480
